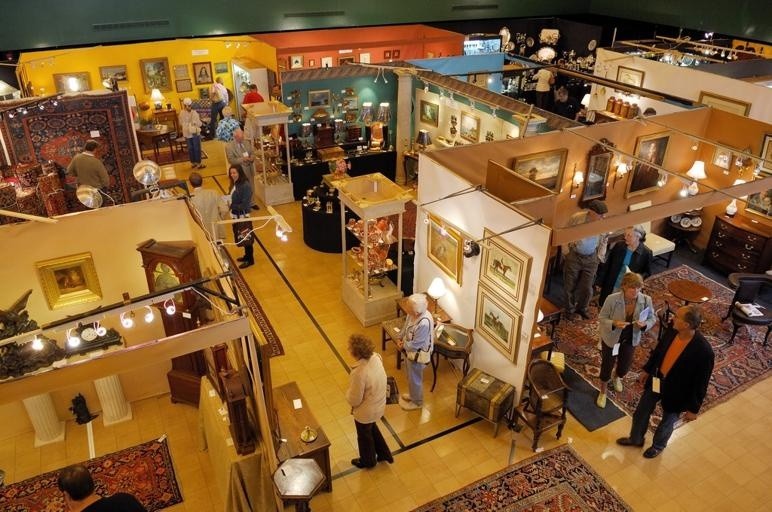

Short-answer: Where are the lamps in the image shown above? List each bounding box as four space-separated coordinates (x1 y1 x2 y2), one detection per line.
76 184 115 208
7 95 62 119
416 129 431 151
29 296 175 350
150 89 164 109
693 44 736 61
273 218 293 242
133 160 162 200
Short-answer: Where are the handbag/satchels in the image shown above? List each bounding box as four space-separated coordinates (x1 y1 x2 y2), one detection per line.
386 376 400 405
235 224 253 247
406 348 430 365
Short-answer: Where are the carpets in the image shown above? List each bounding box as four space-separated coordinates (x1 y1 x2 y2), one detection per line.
542 264 772 436
0 435 184 512
409 442 634 512
560 364 627 433
143 147 208 166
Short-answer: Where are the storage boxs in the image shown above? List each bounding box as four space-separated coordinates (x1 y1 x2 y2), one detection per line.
455 367 515 424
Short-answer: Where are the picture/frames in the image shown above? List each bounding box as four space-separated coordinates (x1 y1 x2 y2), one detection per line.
691 94 756 125
308 89 331 109
420 99 480 143
712 147 738 172
51 56 228 95
426 211 533 365
582 138 613 204
290 53 371 68
635 132 671 194
614 64 647 100
35 252 103 312
513 154 568 202
746 175 772 218
759 134 772 177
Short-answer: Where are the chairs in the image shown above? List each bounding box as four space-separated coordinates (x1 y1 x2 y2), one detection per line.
523 359 571 429
721 277 772 347
628 200 676 269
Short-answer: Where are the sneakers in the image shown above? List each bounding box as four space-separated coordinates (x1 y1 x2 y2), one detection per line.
402 393 410 401
403 402 422 410
377 455 393 463
237 257 243 261
239 262 249 268
613 377 622 393
597 392 606 409
351 458 362 468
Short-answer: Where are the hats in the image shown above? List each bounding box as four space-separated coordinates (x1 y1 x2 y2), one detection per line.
589 199 609 214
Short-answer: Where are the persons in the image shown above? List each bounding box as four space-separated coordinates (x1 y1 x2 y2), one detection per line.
563 200 714 458
394 293 434 410
189 173 229 240
225 164 255 269
57 464 148 512
225 129 260 211
67 140 109 210
346 332 393 469
533 58 656 120
178 76 282 169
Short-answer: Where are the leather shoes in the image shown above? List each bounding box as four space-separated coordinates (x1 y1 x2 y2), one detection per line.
617 438 644 447
644 447 661 458
566 311 592 320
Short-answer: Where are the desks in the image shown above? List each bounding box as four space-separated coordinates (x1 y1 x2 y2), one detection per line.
511 405 566 452
272 380 333 493
665 220 702 254
198 377 278 511
668 280 712 315
430 323 474 393
136 129 174 162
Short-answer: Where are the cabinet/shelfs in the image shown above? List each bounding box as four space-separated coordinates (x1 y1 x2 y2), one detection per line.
231 58 270 123
380 292 452 370
239 102 295 207
330 172 412 329
292 134 397 253
702 212 772 275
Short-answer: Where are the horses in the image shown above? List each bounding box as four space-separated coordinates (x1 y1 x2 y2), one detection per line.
489 311 504 332
492 259 512 277
